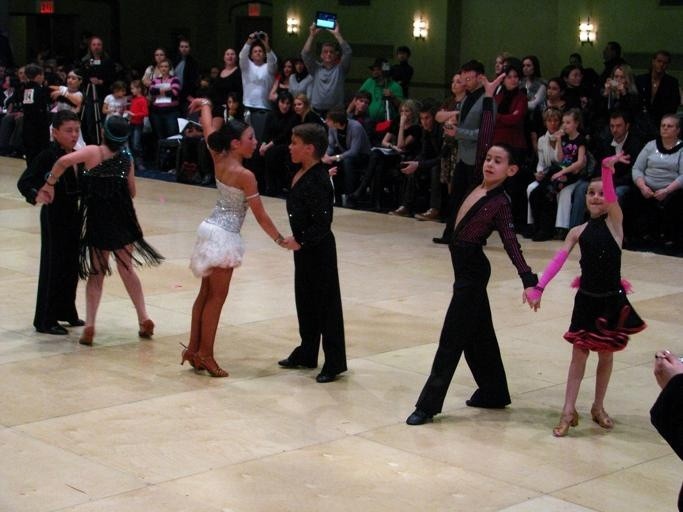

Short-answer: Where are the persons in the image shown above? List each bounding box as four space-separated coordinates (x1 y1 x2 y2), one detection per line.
181 95 287 376
40 116 165 346
527 151 648 437
434 42 682 257
278 122 348 383
406 72 539 425
650 350 683 512
17 110 85 335
2 20 442 223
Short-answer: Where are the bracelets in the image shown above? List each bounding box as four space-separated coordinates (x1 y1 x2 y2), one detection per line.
535 287 544 293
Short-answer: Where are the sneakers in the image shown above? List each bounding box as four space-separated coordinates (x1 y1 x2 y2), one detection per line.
389 206 438 220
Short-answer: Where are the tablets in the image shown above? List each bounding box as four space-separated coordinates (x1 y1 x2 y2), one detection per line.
315 10 337 30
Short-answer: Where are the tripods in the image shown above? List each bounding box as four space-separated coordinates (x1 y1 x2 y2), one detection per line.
77 78 104 145
374 77 401 122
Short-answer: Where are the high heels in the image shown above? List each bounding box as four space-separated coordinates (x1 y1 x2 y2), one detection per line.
591 404 612 428
181 348 228 377
139 320 153 337
554 410 578 436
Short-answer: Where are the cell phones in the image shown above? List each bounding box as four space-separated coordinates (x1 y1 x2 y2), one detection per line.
443 123 454 132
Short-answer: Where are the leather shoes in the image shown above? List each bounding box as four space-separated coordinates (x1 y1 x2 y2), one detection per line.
433 238 449 244
278 359 336 382
407 400 504 425
37 318 94 345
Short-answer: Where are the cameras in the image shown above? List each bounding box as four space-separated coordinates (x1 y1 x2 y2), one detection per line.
88 57 101 70
380 58 393 75
252 31 267 39
